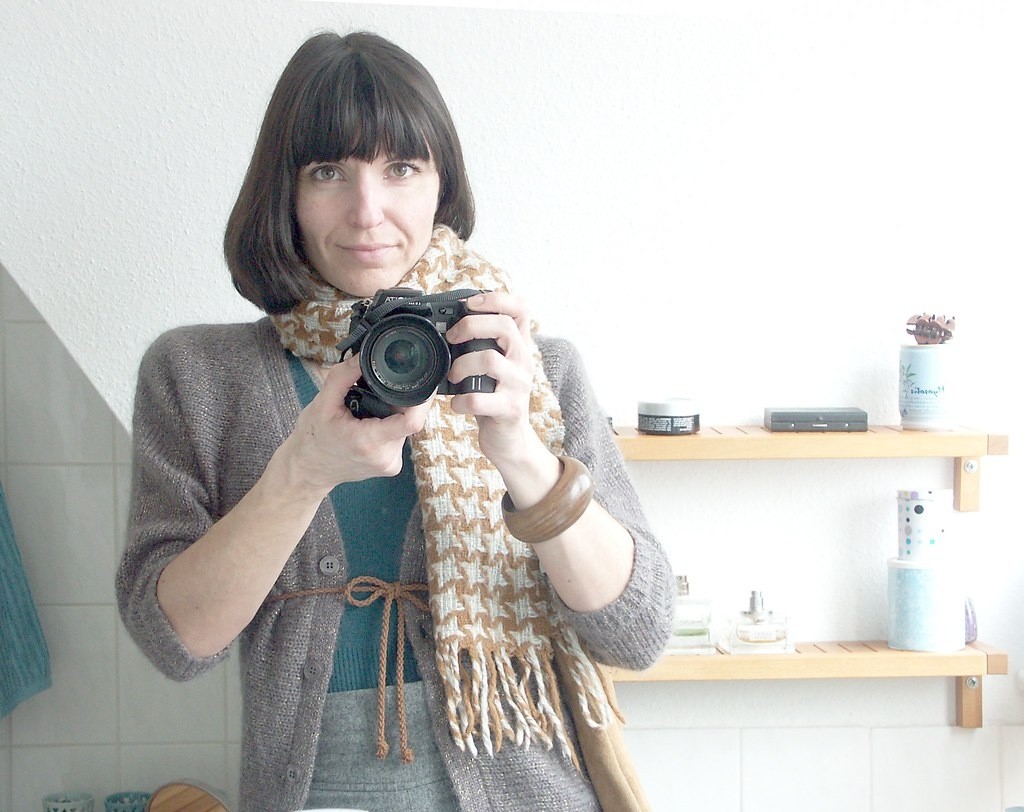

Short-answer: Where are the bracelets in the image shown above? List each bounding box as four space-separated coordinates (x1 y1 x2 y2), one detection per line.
499 454 596 544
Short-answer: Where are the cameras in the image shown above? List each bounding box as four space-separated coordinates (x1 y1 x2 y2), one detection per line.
339 287 504 407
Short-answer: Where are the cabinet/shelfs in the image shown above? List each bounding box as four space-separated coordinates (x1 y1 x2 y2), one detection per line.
610 420 1010 732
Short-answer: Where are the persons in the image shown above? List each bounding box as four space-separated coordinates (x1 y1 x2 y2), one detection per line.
114 30 677 812
